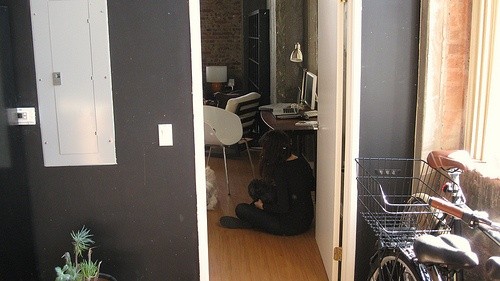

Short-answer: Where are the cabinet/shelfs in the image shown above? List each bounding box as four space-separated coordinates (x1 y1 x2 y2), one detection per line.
247 8 270 105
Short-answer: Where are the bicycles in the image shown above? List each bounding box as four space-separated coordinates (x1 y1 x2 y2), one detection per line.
354 156 500 281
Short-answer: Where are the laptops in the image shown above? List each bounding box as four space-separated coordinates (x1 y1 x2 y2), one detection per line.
273 86 301 116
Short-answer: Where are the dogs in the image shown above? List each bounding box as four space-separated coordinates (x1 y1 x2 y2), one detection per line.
247 177 279 211
204 164 220 211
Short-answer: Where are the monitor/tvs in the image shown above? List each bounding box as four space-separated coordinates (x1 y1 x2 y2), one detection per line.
304 72 318 117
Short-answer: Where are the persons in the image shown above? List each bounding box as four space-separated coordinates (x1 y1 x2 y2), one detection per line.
220 130 316 235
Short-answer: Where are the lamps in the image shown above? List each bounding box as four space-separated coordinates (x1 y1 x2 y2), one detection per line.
205 66 227 90
290 42 304 68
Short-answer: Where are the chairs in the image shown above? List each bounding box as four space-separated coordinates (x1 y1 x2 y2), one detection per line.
204 92 261 196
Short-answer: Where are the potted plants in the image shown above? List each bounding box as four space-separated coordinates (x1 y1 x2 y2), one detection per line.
54 225 117 281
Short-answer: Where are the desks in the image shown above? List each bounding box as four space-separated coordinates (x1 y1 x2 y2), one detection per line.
260 110 317 203
